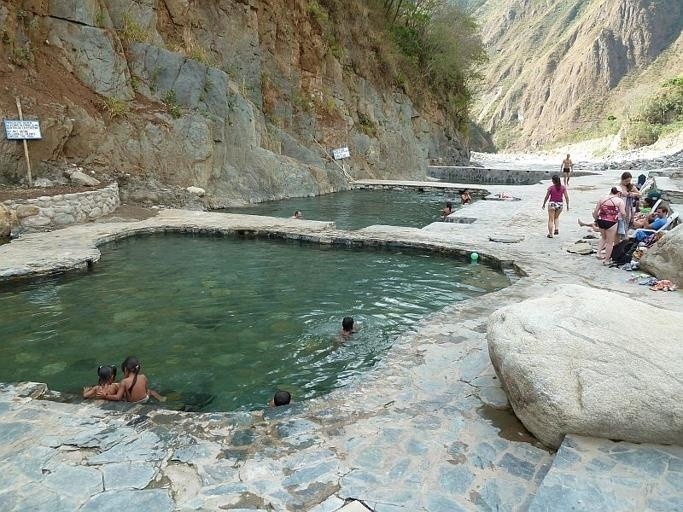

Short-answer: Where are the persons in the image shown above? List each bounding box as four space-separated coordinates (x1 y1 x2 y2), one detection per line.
290 211 303 219
542 174 569 238
443 203 453 217
82 355 168 405
561 154 573 186
578 172 668 266
340 316 355 337
460 188 471 204
268 390 291 408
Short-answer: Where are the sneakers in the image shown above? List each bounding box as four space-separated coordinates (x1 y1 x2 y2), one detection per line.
621 262 640 271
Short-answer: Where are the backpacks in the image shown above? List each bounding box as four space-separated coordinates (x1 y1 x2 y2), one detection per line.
611 238 638 265
